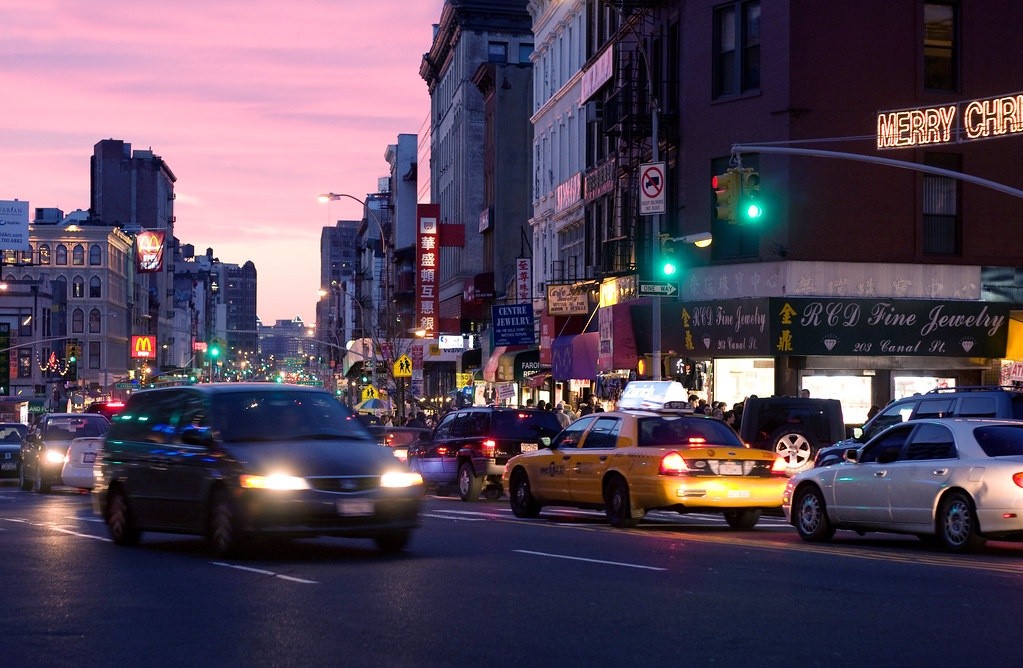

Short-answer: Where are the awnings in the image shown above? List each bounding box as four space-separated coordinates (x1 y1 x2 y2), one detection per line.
498 349 543 381
551 333 601 382
0 396 49 414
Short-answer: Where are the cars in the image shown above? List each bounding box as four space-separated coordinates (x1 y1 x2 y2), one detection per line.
84 400 126 421
782 419 1023 554
60 432 105 489
0 423 29 478
93 382 426 562
501 409 787 528
19 414 111 493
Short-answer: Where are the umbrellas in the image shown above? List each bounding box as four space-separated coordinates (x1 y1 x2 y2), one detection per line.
354 397 398 417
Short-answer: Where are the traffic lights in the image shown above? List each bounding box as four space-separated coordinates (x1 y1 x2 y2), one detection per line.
737 167 762 225
210 339 220 360
64 344 76 366
362 374 369 387
712 170 739 222
76 345 81 360
661 239 678 281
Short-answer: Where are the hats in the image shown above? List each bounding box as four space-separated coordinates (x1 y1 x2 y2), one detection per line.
557 404 562 409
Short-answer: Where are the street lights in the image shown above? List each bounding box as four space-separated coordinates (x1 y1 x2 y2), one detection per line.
653 231 712 382
316 192 391 423
316 289 366 388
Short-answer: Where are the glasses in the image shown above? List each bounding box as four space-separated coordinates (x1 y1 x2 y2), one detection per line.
704 408 711 411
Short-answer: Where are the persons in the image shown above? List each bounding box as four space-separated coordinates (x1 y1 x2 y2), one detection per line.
864 406 881 432
525 394 587 429
580 393 605 420
800 388 811 400
880 397 901 429
405 411 440 430
382 415 394 428
684 395 699 410
697 395 760 429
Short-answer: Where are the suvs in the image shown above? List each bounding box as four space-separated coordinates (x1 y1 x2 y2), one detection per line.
814 387 1023 469
406 405 561 503
739 395 846 469
